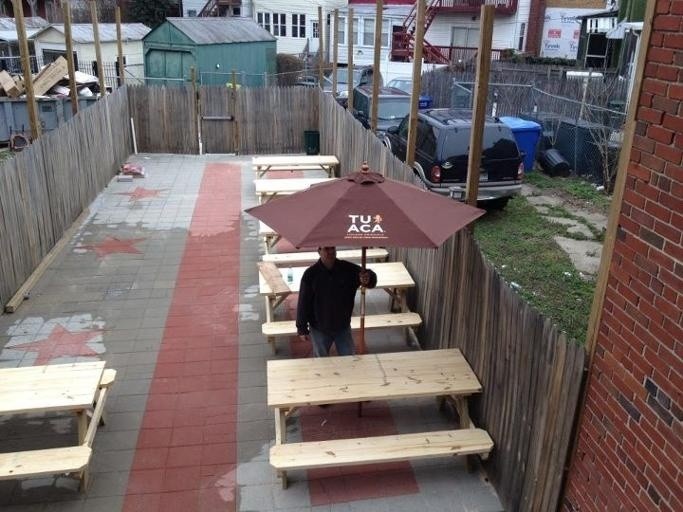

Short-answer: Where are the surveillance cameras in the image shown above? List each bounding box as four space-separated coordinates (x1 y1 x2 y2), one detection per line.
472 14 476 21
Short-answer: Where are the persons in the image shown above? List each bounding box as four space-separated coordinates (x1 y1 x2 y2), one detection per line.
294 245 377 408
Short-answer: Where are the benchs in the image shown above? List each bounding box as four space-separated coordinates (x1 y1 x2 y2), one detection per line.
0 361 117 494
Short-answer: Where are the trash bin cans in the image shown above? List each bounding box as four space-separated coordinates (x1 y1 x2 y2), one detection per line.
499 116 541 173
304 131 319 155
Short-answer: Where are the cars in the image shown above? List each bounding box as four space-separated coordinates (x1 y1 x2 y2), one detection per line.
321 65 383 107
388 77 412 97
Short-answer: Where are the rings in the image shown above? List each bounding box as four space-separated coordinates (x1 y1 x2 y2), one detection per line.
363 279 367 282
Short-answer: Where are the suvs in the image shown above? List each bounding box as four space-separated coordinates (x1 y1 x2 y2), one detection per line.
346 85 410 141
384 107 523 211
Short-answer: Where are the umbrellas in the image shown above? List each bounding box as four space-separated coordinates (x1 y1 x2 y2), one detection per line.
244 160 486 420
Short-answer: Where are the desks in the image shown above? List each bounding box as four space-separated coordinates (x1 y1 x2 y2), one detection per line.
253 155 339 180
